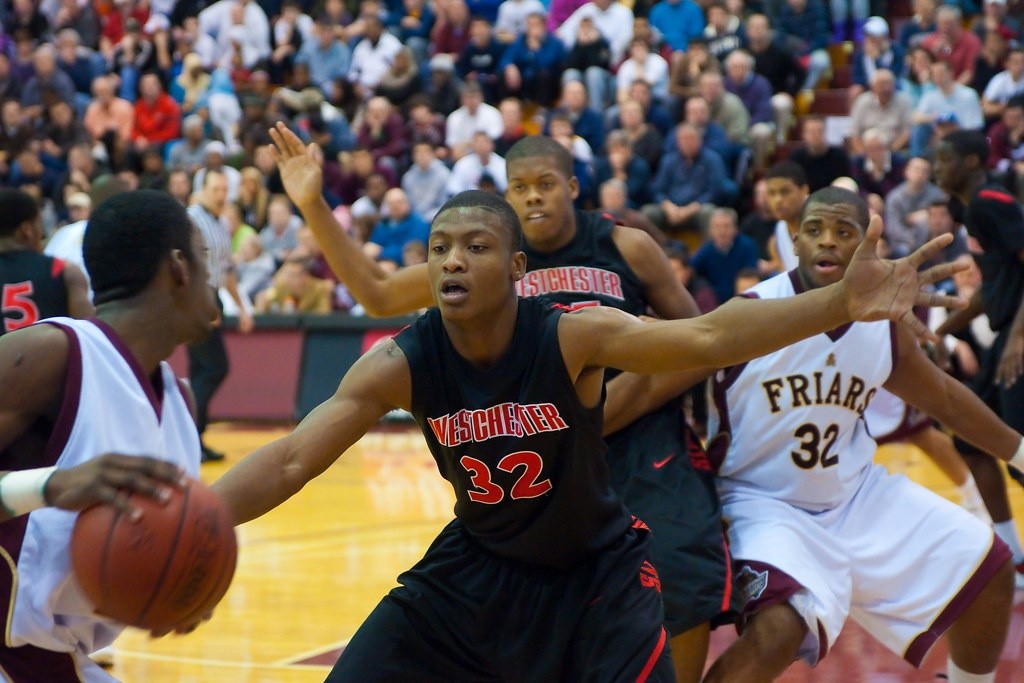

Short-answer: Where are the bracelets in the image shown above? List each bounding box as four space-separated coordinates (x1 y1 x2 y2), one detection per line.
0 464 59 516
1007 437 1024 476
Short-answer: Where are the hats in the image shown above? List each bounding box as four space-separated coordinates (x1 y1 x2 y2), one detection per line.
864 17 890 36
68 193 91 208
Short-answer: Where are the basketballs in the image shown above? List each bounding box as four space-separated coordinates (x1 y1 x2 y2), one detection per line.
71 477 237 631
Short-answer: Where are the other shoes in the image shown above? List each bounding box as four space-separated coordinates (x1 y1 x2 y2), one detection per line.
201 446 225 462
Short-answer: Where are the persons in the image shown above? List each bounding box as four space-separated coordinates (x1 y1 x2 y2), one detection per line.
0 0 1024 682
